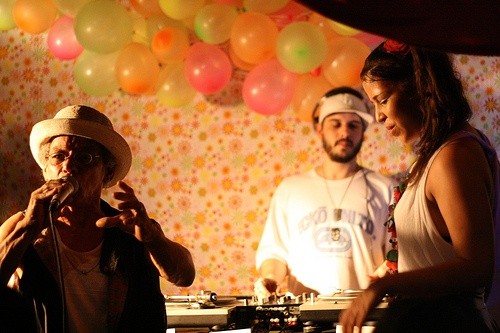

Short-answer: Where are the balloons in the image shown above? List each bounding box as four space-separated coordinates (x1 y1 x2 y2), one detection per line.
0 0 381 115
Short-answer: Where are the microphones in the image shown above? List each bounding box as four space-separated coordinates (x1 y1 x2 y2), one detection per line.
50 175 79 210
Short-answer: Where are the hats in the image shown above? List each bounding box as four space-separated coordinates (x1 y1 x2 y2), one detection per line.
28 105 132 191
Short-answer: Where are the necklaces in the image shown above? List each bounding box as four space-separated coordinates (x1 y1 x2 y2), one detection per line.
385 171 411 273
322 164 357 220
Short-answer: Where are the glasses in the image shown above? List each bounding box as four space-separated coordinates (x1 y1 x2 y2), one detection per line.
43 151 105 167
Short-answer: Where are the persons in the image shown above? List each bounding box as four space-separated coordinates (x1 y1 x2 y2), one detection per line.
0 105 196 333
340 40 500 333
255 86 398 333
